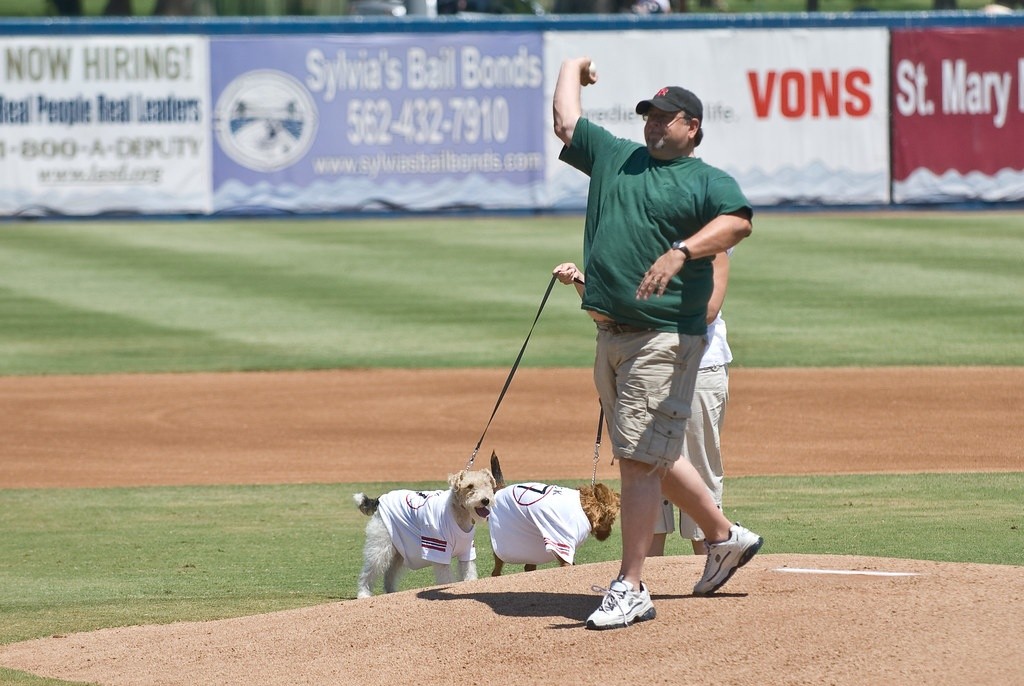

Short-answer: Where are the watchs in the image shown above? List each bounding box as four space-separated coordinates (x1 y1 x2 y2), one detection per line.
671 240 692 262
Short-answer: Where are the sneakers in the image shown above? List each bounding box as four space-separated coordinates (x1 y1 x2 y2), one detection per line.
694 521 764 594
586 575 656 629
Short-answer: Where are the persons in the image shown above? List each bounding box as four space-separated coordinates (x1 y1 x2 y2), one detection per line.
553 56 763 629
552 245 733 557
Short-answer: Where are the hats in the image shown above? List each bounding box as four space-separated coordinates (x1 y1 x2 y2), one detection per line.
636 85 703 125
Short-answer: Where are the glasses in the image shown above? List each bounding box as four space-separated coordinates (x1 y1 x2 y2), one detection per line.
642 111 693 125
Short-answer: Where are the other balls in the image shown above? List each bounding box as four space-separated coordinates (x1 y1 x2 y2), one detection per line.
589 60 597 77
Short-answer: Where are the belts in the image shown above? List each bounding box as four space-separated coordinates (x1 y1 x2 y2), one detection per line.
595 320 645 335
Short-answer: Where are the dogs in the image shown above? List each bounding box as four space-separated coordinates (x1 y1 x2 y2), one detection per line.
353 469 496 600
487 450 621 577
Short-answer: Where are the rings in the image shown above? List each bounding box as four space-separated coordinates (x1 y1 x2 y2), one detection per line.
653 278 658 282
